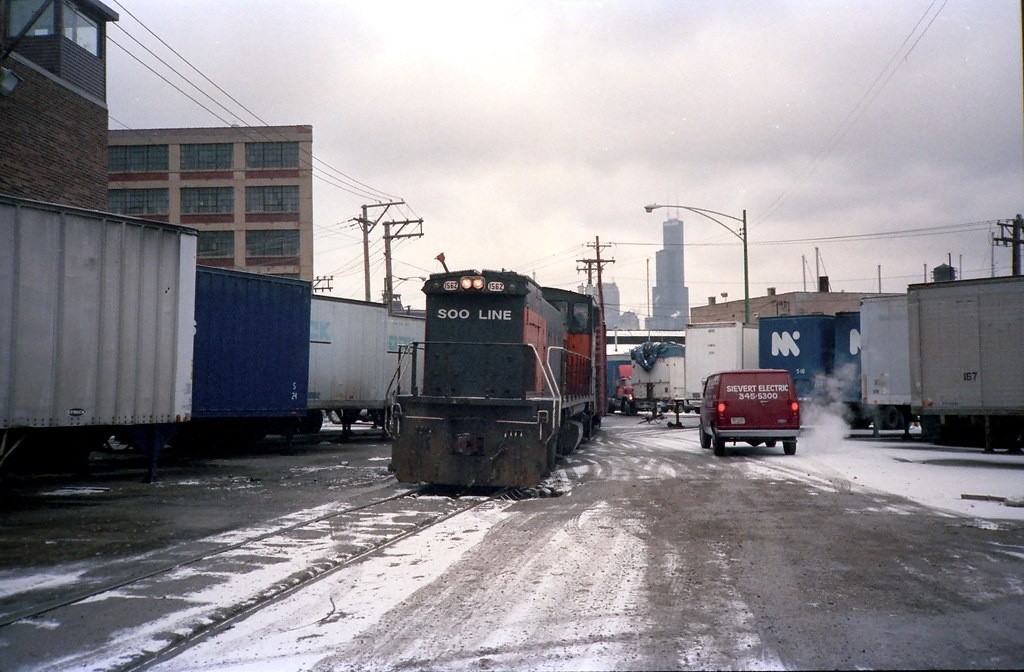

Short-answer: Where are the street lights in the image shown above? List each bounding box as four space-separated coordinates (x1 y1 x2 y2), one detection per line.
644 200 753 322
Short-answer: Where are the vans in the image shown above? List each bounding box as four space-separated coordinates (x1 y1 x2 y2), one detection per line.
703 370 801 455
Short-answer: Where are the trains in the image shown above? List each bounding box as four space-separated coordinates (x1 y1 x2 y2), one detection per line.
390 269 608 499
